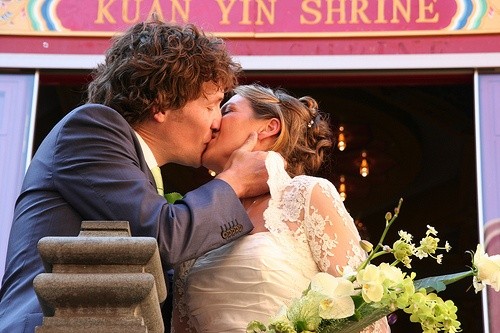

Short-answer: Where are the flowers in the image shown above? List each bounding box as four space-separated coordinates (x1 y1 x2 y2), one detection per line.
246 195 500 333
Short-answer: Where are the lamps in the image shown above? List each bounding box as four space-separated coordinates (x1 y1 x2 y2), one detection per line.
339 176 348 201
359 148 370 178
337 125 347 151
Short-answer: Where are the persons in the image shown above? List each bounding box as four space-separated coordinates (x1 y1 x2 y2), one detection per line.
165 82 392 333
0 10 287 333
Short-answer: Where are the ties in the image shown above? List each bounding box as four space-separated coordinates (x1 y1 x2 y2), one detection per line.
151 167 163 197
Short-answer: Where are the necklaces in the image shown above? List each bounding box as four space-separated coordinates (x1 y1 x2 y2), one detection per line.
242 197 258 214
